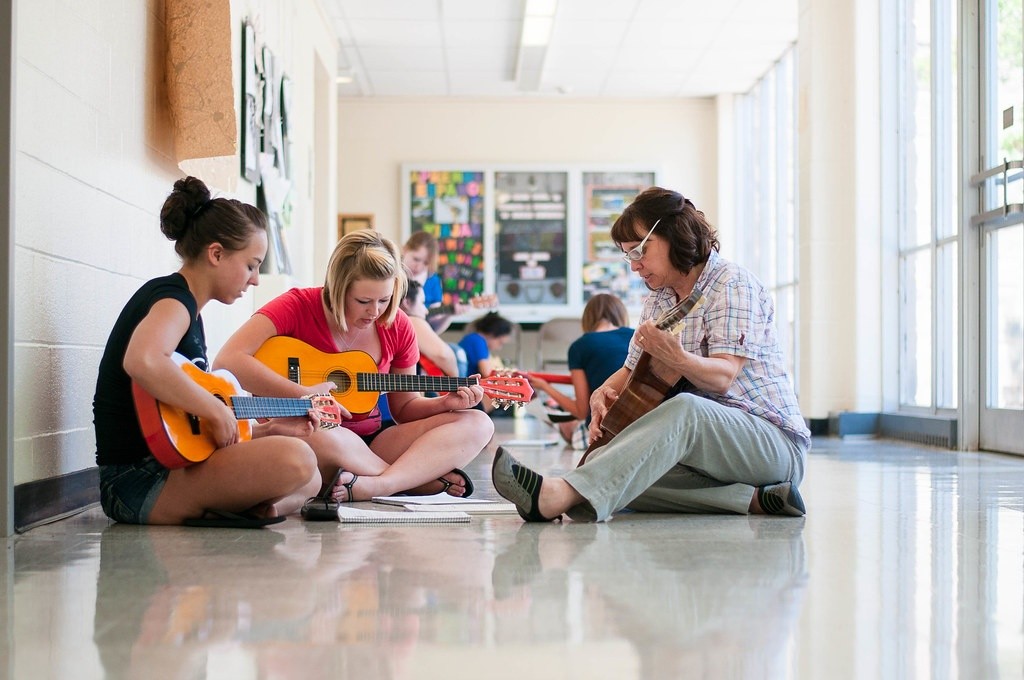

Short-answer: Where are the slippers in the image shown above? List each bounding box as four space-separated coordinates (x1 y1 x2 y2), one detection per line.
323 468 358 502
183 509 286 528
438 468 474 498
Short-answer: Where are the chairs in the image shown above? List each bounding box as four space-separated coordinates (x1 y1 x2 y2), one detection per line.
465 319 523 370
533 319 585 404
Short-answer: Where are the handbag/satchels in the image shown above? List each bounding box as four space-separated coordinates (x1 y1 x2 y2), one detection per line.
301 497 339 521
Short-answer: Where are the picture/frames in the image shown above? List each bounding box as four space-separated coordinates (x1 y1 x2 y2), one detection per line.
241 23 262 187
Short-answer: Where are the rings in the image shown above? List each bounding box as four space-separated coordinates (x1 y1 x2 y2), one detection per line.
639 336 644 342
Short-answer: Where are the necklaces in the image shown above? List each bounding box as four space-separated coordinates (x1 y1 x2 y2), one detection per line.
336 326 362 351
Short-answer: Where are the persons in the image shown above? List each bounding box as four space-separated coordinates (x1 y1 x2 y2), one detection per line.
92 176 323 525
526 294 635 450
213 230 495 502
400 232 523 413
492 188 811 521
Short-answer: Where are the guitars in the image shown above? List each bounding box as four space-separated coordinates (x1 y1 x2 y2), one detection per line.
131 349 343 469
252 333 535 425
489 367 574 386
427 290 498 319
577 288 707 467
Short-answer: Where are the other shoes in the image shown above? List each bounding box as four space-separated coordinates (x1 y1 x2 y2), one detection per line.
492 446 563 523
758 481 806 516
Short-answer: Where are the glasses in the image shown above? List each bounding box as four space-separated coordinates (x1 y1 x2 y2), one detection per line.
624 219 661 264
